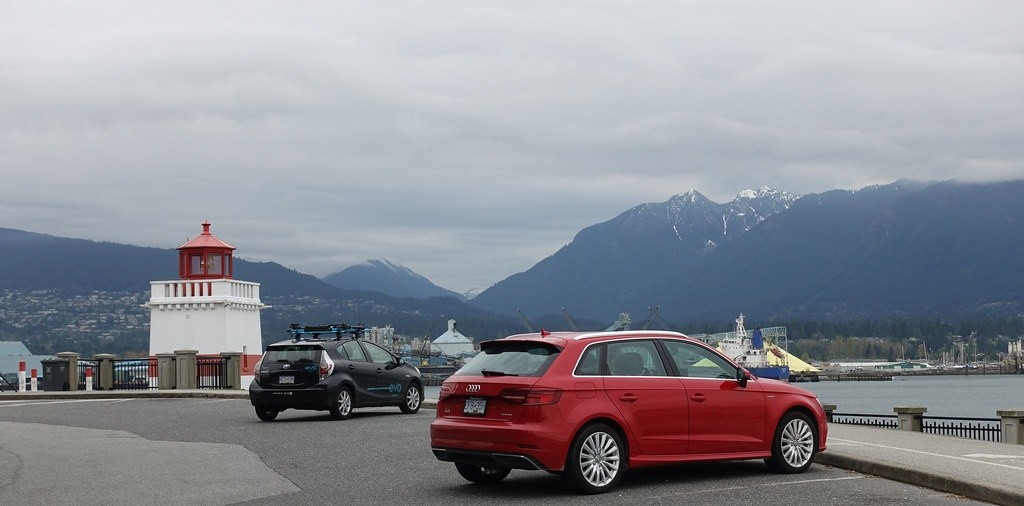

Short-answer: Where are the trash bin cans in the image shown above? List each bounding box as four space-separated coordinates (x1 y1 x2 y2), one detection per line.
40 358 71 391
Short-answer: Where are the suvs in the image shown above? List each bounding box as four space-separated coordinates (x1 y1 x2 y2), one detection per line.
249 324 425 421
430 329 830 495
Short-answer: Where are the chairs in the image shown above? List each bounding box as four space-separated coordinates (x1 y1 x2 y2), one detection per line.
611 352 643 376
341 346 353 359
580 353 598 376
